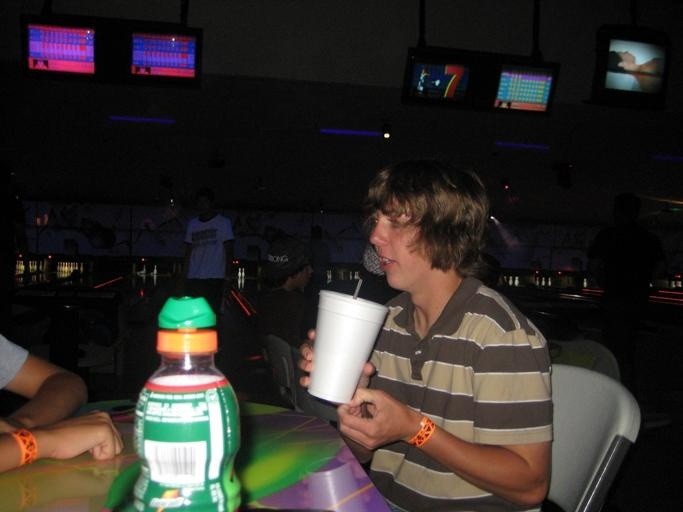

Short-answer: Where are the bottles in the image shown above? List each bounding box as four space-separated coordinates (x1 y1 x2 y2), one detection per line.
130 297 243 512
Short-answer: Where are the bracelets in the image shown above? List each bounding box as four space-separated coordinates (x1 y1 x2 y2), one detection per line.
406 416 437 448
11 428 37 468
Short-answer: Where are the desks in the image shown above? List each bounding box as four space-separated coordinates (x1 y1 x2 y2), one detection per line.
0 397 393 512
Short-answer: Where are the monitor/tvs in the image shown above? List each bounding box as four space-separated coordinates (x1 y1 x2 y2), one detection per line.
410 62 471 102
26 24 96 75
129 32 198 79
492 64 553 113
590 24 672 110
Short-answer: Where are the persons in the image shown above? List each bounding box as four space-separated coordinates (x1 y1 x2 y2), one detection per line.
259 242 318 348
586 191 665 363
0 334 124 475
183 187 235 314
0 161 31 337
300 155 553 512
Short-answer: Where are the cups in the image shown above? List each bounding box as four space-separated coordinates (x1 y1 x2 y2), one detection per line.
305 456 365 512
304 288 389 404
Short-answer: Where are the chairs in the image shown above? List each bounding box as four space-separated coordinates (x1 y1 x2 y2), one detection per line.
258 332 297 408
290 346 338 424
546 364 642 512
550 339 672 431
